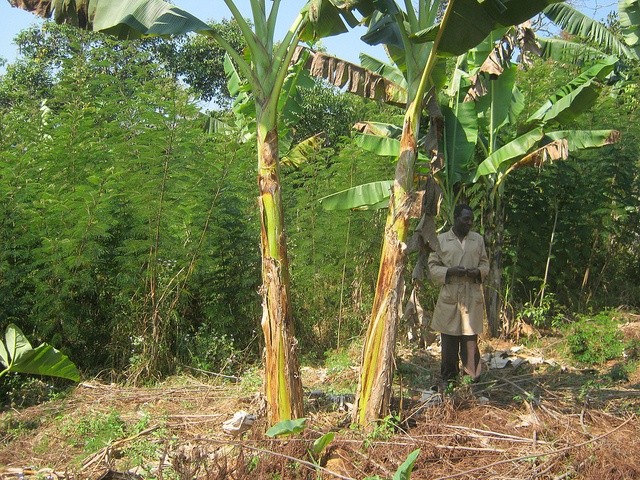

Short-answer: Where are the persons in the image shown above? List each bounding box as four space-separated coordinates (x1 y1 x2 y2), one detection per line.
428 204 489 383
